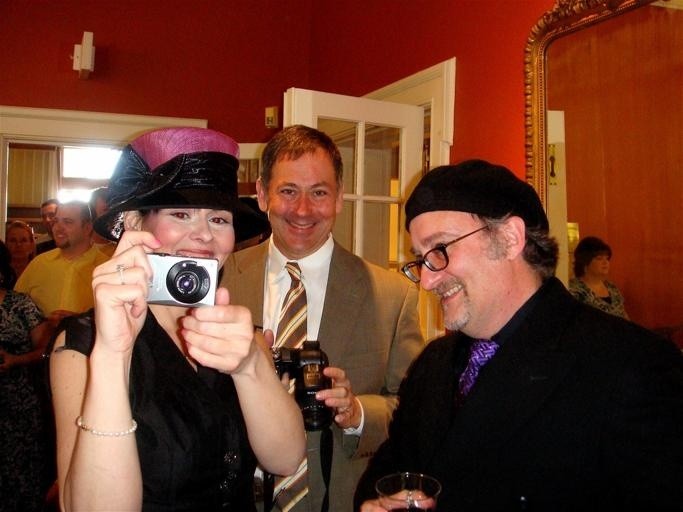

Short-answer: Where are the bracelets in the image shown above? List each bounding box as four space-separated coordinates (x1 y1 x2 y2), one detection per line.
74 415 140 441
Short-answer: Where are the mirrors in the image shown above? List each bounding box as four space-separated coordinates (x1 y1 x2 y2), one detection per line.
522 0 682 350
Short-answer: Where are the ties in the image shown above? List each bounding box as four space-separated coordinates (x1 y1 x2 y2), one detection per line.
270 261 310 511
457 339 500 396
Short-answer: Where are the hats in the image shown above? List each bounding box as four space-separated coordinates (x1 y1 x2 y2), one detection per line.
404 159 549 235
92 126 268 248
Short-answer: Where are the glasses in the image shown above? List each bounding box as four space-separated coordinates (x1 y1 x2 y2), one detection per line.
400 225 490 284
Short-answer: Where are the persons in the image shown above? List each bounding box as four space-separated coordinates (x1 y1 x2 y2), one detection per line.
1 238 51 512
46 126 307 512
351 156 682 512
214 124 427 512
0 187 117 289
568 235 638 323
10 201 121 321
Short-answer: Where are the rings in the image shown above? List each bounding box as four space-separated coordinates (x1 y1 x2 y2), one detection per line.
114 261 127 274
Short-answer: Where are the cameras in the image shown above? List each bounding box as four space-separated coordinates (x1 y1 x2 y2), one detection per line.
270 341 336 433
142 252 218 309
